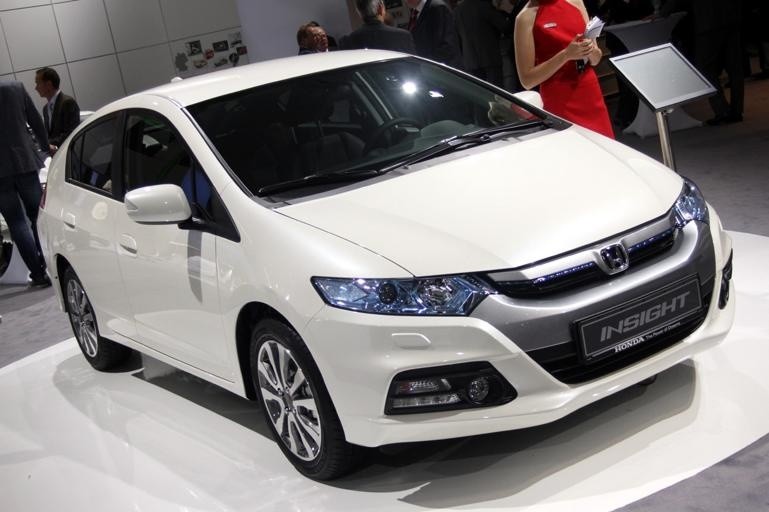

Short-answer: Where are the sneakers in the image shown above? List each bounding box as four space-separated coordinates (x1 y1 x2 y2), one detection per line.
28 273 52 288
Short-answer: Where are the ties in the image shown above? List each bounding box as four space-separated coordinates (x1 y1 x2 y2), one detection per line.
410 9 419 32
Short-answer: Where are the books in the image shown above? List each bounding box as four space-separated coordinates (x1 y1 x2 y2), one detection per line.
576 16 606 71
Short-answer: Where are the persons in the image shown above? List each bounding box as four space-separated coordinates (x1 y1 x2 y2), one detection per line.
749 0 769 81
0 79 59 290
35 66 80 152
406 0 464 72
494 1 539 92
597 0 654 129
514 0 615 144
296 21 337 53
453 0 515 92
643 0 749 127
338 0 416 55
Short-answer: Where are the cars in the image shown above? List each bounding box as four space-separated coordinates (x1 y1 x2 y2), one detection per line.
37 48 736 481
0 110 97 286
